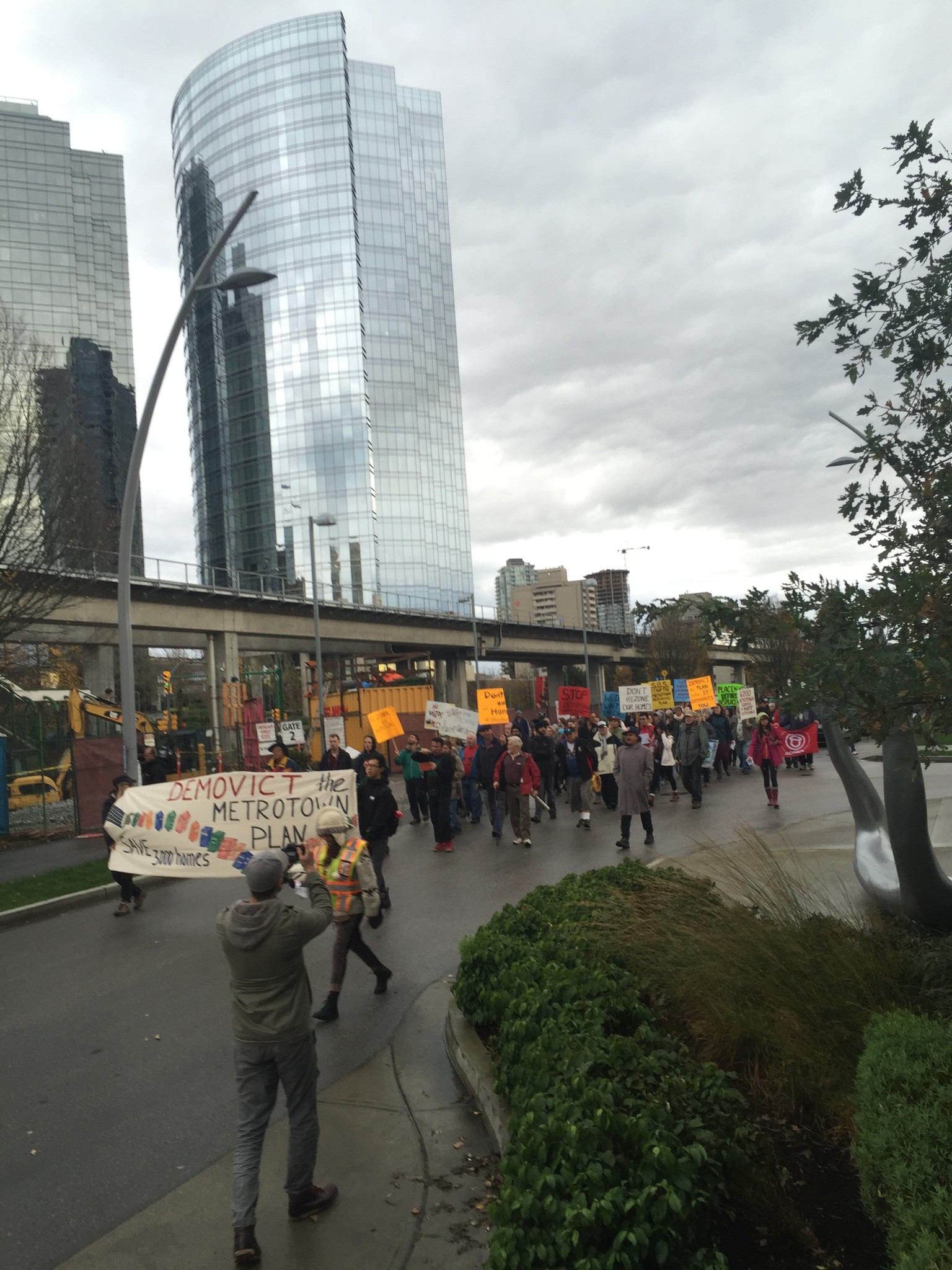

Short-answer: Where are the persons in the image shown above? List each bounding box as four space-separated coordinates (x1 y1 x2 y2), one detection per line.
394 695 857 849
356 751 402 911
101 774 145 916
319 733 351 770
213 840 336 1267
309 806 393 1022
353 735 389 783
263 741 299 772
142 745 167 785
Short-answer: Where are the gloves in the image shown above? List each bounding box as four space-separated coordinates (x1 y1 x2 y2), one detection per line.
367 910 384 930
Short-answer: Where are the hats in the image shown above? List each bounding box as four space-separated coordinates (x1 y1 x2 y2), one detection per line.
244 848 291 893
621 726 641 742
755 712 769 725
683 709 697 717
481 725 492 732
597 720 608 728
268 741 289 757
563 726 575 735
609 716 620 723
112 774 138 787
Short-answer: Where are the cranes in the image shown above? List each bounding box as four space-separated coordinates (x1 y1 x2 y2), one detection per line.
616 546 649 571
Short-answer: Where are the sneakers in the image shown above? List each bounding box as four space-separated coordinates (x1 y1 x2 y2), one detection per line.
231 1224 262 1266
615 837 630 849
113 901 131 916
691 790 703 809
576 818 591 828
133 887 147 911
644 833 655 844
431 840 455 852
512 837 533 849
288 1183 339 1222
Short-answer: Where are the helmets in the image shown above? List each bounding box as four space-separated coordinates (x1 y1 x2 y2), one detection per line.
314 806 355 847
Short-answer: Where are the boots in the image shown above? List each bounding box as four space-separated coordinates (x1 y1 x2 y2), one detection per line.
310 990 340 1022
647 793 655 806
670 789 680 802
372 961 394 996
764 785 779 808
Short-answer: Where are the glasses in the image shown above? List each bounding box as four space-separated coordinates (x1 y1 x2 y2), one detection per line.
639 718 648 721
363 761 381 769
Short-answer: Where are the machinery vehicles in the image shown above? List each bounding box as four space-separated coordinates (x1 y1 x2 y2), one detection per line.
8 748 71 812
69 685 202 782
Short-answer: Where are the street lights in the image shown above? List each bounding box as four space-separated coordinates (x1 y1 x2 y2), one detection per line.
117 190 278 790
457 594 482 689
578 578 599 690
309 514 339 752
